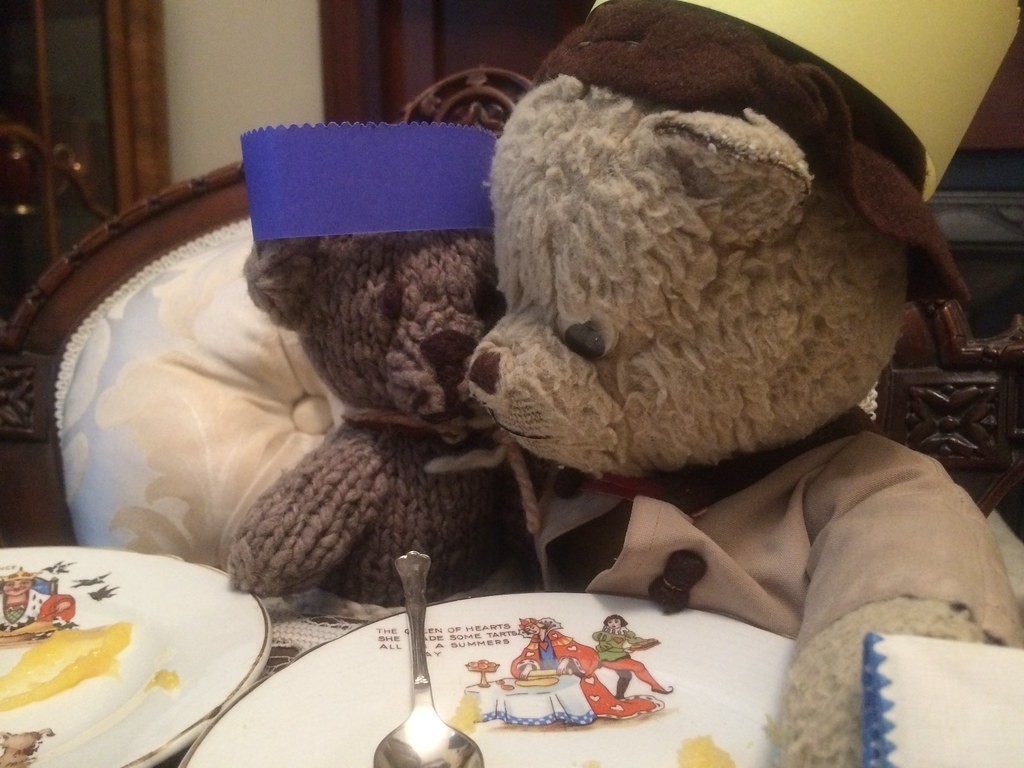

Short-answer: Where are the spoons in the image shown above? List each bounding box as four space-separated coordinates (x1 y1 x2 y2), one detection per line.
372 550 484 768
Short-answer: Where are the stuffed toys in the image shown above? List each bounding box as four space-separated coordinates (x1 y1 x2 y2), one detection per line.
226 226 544 610
466 0 1024 768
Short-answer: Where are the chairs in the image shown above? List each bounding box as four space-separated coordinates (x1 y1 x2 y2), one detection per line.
0 64 1023 572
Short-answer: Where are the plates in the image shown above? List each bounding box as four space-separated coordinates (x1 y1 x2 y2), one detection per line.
1 542 272 768
174 590 795 768
